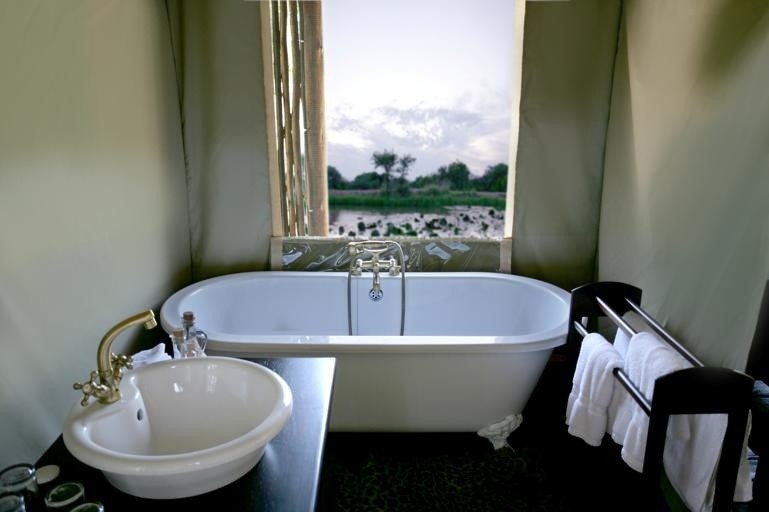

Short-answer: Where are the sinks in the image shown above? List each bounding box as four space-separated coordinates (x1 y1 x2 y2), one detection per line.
63 356 293 500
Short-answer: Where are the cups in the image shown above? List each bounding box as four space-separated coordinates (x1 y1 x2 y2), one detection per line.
0 462 41 511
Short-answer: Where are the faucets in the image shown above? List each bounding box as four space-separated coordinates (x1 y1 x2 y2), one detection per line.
354 254 402 300
73 306 157 405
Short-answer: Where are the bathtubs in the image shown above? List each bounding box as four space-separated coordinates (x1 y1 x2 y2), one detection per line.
160 267 571 433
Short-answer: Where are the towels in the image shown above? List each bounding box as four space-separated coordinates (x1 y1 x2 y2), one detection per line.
563 311 754 512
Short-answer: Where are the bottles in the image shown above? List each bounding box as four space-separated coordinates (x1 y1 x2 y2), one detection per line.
169 310 208 360
